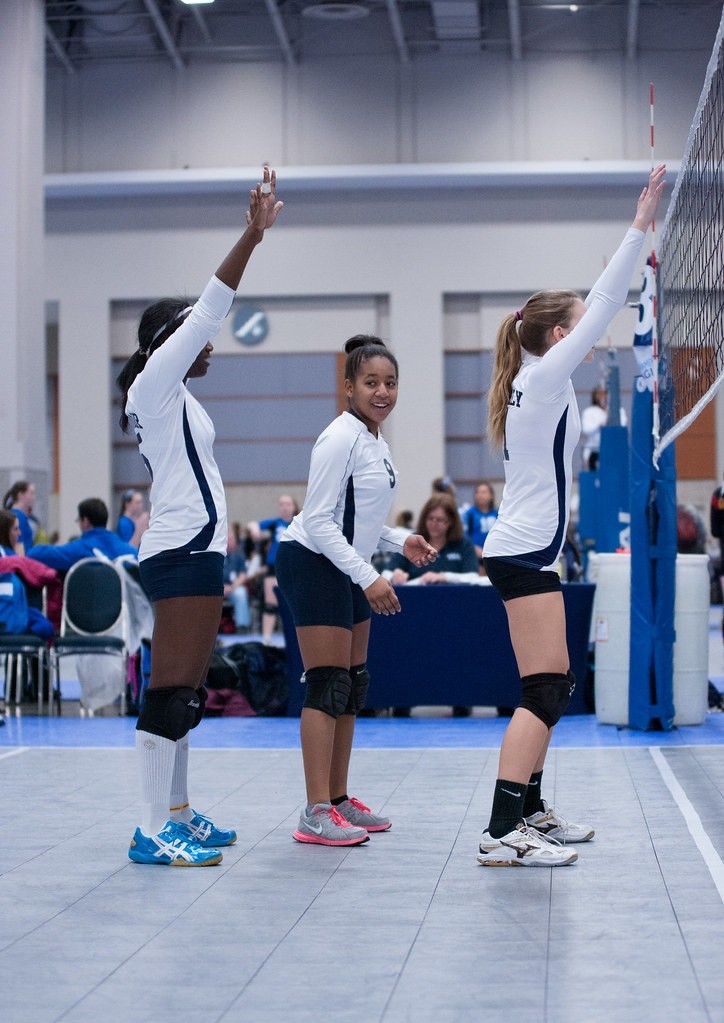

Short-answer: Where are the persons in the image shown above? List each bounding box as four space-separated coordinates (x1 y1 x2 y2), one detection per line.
116 165 282 867
479 166 667 868
0 476 499 699
582 384 628 469
274 333 438 846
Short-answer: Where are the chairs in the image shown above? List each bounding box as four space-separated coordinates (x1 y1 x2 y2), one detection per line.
0 554 155 718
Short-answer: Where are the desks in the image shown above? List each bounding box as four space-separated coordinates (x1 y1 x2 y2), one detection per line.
272 583 596 716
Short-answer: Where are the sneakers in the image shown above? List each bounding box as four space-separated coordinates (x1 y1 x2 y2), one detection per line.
130 821 222 866
478 819 579 867
177 810 237 847
334 797 392 830
524 800 595 843
292 804 370 846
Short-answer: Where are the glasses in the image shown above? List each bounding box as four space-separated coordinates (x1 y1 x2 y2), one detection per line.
424 515 450 524
